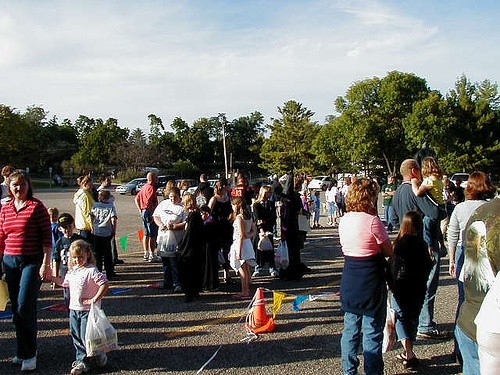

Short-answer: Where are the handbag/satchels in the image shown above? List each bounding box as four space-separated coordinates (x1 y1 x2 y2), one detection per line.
85 299 117 357
275 240 289 270
258 236 273 251
160 229 178 254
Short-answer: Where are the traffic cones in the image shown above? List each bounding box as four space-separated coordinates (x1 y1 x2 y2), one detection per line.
245 288 271 328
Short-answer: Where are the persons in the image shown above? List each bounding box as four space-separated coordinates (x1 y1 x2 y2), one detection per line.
45 238 112 374
386 210 434 369
133 170 162 263
411 157 448 257
0 156 500 375
380 173 397 235
309 191 322 228
227 196 256 299
251 185 279 278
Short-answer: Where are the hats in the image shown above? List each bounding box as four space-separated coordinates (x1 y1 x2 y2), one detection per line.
57 213 74 226
387 174 395 178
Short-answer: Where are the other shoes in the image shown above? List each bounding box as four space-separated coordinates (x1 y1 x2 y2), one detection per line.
252 271 261 277
173 286 182 293
317 224 321 227
13 356 22 363
313 225 318 228
395 353 416 367
328 221 332 225
332 222 337 227
71 361 87 375
232 294 250 299
148 255 161 263
271 271 278 277
98 355 107 365
416 332 446 343
21 350 37 370
113 259 124 264
143 255 149 260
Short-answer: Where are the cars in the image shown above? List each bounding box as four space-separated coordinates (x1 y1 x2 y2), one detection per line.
135 175 220 197
306 175 336 191
115 178 147 195
450 172 470 189
